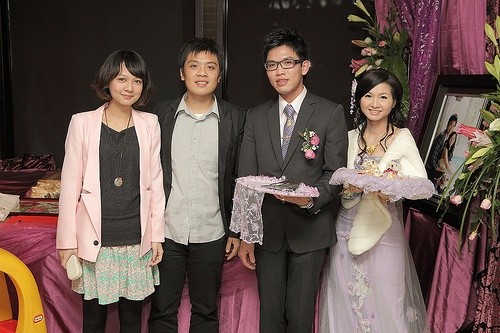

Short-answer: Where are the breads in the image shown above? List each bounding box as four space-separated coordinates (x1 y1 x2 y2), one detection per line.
29 179 61 198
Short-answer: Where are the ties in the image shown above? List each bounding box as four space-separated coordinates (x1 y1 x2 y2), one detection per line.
281 104 296 165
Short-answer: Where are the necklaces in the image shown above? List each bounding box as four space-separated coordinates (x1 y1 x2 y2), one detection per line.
105 108 132 187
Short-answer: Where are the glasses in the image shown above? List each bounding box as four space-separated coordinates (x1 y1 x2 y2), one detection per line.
264 58 304 71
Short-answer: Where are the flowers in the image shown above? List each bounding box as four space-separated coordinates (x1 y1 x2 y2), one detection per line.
434 15 500 255
347 0 414 128
297 128 320 160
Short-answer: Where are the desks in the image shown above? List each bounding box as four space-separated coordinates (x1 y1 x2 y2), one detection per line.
0 169 261 333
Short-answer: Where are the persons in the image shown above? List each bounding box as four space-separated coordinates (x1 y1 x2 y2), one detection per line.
147 37 246 333
56 49 164 333
426 114 458 194
317 69 431 333
238 26 348 333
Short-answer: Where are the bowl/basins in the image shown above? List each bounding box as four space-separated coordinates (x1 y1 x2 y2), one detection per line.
0 166 47 198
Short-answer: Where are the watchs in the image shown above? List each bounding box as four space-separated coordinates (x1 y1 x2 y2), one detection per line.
300 197 314 209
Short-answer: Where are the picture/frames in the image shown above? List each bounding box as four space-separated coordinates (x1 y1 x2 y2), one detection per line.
401 74 500 234
